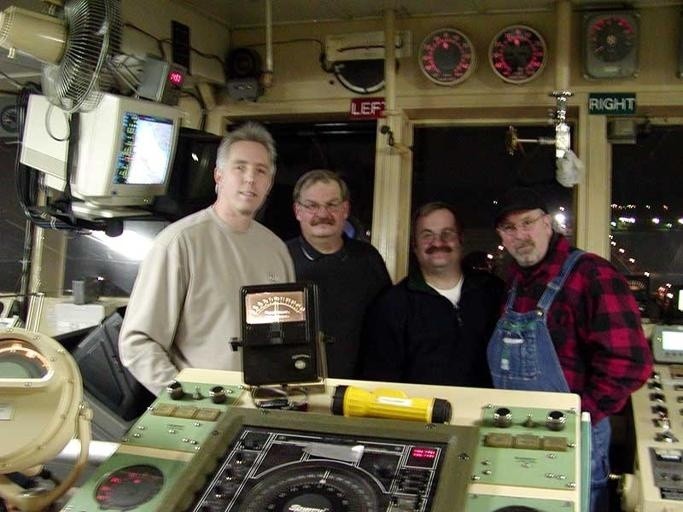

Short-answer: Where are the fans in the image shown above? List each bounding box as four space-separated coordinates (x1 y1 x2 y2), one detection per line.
0 1 126 113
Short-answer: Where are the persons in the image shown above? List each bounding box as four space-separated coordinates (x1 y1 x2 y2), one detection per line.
479 183 654 512
282 165 392 378
116 115 298 397
357 199 508 386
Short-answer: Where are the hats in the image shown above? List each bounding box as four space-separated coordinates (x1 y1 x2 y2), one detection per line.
492 188 549 219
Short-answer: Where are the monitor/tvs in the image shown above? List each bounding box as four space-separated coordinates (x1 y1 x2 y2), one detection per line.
19 91 182 219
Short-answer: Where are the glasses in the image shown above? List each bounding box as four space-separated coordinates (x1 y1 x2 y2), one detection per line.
416 230 461 243
297 200 346 212
499 215 544 234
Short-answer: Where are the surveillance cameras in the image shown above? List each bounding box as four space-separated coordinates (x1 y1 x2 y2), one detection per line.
378 114 393 146
